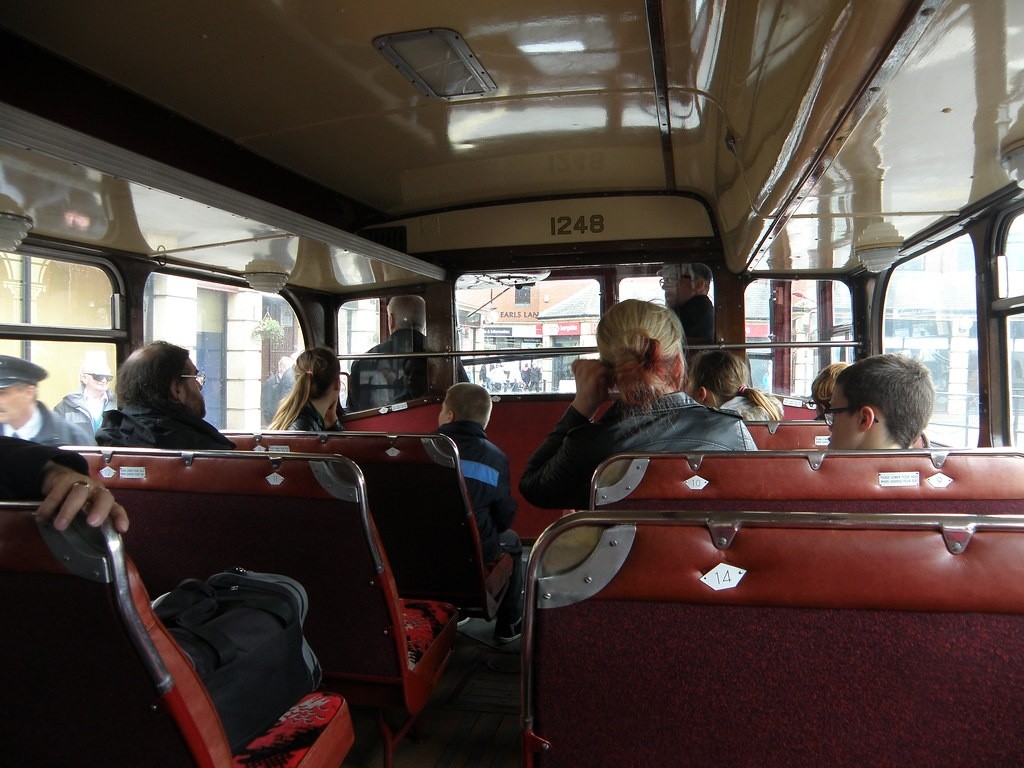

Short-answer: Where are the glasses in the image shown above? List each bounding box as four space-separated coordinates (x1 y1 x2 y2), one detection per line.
168 372 207 388
805 397 830 411
84 372 114 383
822 406 880 428
660 272 698 288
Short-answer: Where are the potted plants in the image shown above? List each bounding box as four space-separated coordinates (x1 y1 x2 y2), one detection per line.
252 318 285 343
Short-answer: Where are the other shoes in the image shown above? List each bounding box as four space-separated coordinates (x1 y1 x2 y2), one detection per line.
493 616 526 644
452 607 470 626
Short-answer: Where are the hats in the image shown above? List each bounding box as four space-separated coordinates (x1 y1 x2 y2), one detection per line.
0 354 47 389
657 260 712 282
82 351 114 376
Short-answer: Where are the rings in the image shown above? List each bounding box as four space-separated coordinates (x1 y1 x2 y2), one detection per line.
73 479 90 496
94 486 110 493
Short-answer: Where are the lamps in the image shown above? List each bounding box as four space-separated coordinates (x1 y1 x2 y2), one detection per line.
853 221 904 274
0 210 33 254
242 258 291 296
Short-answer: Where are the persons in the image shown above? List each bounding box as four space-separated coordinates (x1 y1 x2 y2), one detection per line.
266 347 347 432
659 263 714 345
687 350 785 420
519 300 758 510
93 341 236 450
0 434 128 534
54 363 117 437
811 350 934 453
479 363 540 392
0 355 90 446
435 382 515 567
345 294 470 413
262 352 296 425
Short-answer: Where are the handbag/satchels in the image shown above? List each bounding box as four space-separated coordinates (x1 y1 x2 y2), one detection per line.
538 373 542 380
156 565 324 756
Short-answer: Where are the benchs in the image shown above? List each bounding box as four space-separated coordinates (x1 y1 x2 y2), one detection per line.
0 421 1024 768
